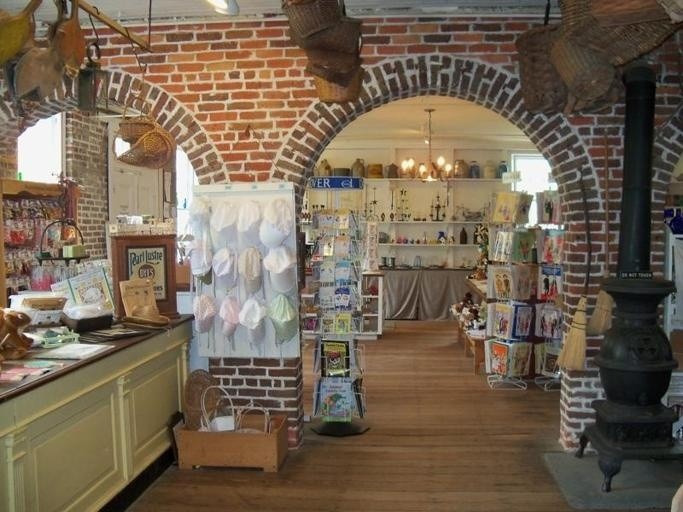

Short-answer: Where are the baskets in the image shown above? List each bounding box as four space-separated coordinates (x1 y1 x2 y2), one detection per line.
113 96 177 170
516 1 683 118
280 1 363 105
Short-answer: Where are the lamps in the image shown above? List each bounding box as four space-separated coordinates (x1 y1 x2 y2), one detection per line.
208 0 239 16
401 109 458 181
77 43 112 110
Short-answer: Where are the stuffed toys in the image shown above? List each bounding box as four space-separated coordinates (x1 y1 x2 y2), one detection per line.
0 306 34 361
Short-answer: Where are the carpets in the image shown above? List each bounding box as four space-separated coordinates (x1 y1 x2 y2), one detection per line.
543 453 683 510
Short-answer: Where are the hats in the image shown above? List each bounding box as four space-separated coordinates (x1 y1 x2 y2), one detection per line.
189 198 297 335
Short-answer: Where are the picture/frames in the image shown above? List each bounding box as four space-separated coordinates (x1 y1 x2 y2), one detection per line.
110 235 181 319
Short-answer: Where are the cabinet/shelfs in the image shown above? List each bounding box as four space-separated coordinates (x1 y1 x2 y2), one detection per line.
304 177 512 268
487 188 565 394
451 234 486 372
305 180 370 432
0 180 77 308
303 272 388 342
0 314 195 512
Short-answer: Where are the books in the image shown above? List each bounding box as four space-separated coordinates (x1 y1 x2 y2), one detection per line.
15 267 163 349
479 187 568 379
306 212 368 422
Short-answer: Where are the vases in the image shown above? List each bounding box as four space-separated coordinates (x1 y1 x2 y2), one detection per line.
318 156 383 178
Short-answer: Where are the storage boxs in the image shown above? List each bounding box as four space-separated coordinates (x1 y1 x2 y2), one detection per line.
173 414 289 472
